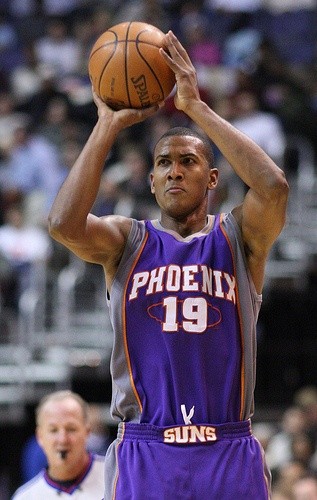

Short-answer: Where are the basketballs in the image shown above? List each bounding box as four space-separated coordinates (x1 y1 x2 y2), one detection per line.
89 21 176 110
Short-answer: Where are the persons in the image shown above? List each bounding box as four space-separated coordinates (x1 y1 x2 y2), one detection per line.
10 390 106 500
45 30 288 500
253 388 317 500
0 0 317 341
